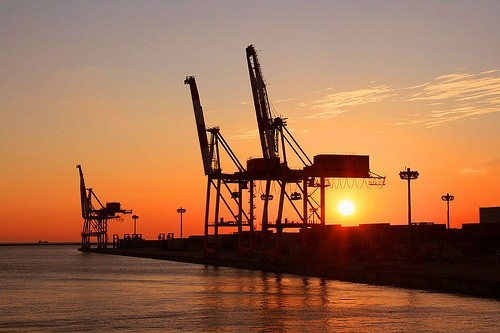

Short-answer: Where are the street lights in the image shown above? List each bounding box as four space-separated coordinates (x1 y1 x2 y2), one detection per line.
177 207 186 238
132 214 140 238
441 193 455 230
399 166 419 224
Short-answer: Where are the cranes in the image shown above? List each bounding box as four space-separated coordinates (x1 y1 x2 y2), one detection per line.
183 75 253 253
75 164 133 252
240 43 387 256
